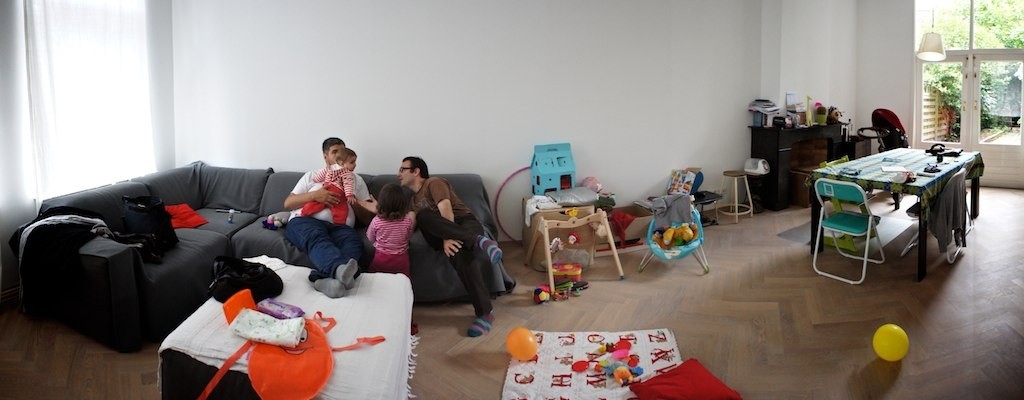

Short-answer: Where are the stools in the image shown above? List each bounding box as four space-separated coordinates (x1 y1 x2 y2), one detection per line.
714 170 754 223
787 164 822 208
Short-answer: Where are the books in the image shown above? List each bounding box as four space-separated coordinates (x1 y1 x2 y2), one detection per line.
749 98 782 127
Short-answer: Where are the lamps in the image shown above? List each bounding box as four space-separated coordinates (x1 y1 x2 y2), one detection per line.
917 0 947 62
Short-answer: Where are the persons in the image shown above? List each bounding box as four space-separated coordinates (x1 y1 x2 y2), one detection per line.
295 148 358 232
282 136 371 299
364 183 419 336
357 156 504 337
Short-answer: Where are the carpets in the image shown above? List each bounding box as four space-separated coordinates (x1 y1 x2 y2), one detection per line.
775 211 915 259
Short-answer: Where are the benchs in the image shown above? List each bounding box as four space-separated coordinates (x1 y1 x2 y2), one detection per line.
156 256 414 400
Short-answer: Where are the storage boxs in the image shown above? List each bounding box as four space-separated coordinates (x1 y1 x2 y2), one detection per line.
595 203 653 246
521 195 596 271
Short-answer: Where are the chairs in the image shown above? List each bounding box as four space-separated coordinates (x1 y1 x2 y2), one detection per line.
636 201 710 277
958 154 974 239
820 153 878 252
812 177 886 286
896 168 967 263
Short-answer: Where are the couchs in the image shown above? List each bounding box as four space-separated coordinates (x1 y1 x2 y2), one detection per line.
231 169 505 304
40 159 273 357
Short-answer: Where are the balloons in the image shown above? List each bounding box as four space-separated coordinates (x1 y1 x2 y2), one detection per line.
506 326 539 362
872 324 911 364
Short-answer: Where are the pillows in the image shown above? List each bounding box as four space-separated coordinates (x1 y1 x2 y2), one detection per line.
629 357 744 400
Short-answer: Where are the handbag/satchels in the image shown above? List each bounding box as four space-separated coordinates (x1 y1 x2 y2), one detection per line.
117 194 180 253
208 254 283 304
248 317 334 400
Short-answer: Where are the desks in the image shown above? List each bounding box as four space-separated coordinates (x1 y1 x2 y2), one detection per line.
649 189 723 226
808 146 985 283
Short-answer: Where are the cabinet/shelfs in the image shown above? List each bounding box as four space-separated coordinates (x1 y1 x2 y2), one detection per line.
746 122 843 212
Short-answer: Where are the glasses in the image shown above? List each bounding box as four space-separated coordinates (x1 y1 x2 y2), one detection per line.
399 167 415 173
